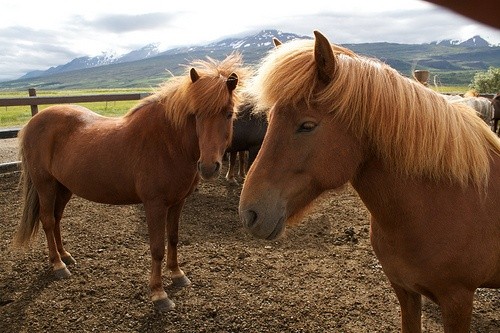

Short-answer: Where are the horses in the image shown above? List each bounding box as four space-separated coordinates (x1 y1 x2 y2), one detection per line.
223 104 269 184
238 28 500 333
488 96 500 133
12 49 255 313
470 97 495 125
